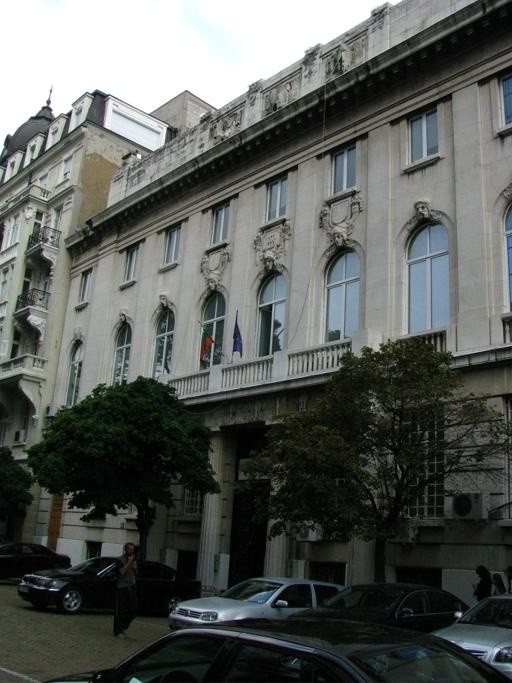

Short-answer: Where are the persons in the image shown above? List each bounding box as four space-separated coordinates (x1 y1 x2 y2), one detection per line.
331 226 347 248
119 312 127 323
158 292 169 308
206 274 220 291
112 541 138 640
493 572 507 595
262 252 277 271
472 565 492 603
413 197 432 221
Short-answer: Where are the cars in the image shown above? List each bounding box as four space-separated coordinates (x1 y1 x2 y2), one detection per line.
0 540 74 580
424 593 511 683
166 573 348 636
41 618 511 683
18 556 203 616
286 579 470 635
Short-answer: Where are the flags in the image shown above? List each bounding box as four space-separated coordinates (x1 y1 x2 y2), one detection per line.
233 320 243 358
199 329 214 359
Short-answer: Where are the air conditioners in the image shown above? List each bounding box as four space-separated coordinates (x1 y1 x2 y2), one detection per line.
451 490 490 522
294 518 324 543
45 403 59 417
14 429 27 443
